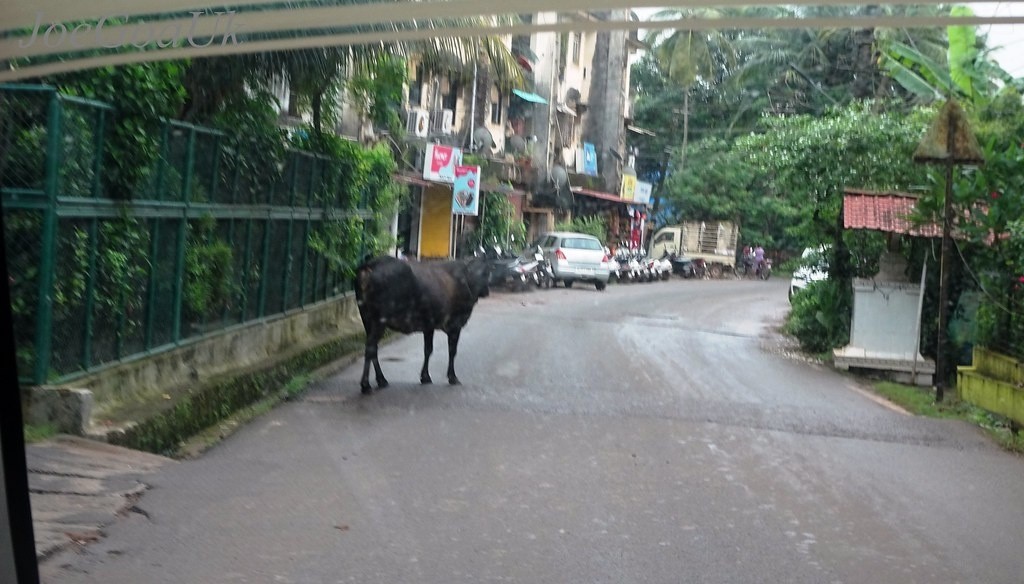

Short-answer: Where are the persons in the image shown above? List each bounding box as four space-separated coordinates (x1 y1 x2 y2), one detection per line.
614 240 630 280
743 242 765 277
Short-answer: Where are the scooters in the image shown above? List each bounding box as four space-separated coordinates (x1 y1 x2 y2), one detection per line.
603 240 673 284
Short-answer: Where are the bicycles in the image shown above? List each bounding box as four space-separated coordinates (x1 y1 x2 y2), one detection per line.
733 259 772 281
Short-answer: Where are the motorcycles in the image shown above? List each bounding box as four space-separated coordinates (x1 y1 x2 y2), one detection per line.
660 243 707 278
474 234 554 293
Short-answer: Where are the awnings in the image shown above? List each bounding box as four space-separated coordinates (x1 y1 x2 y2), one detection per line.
389 167 526 199
570 185 631 204
512 88 548 105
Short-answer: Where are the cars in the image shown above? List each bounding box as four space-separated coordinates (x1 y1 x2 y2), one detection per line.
532 231 610 291
788 243 832 302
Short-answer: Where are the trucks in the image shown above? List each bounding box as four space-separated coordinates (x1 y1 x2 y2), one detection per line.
650 221 739 279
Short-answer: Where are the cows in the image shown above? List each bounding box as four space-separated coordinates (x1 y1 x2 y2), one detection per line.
354 254 498 395
